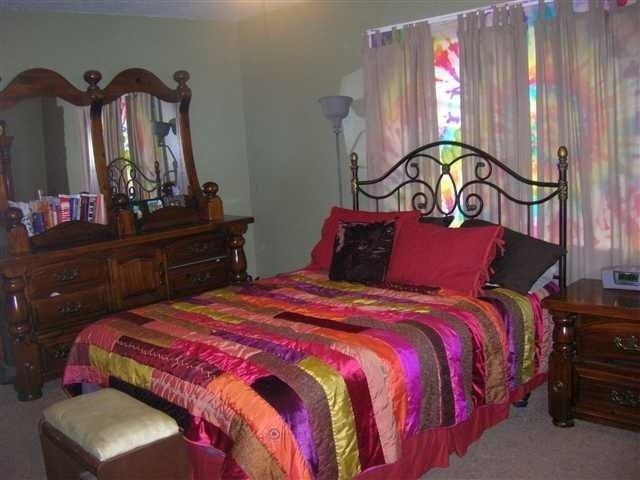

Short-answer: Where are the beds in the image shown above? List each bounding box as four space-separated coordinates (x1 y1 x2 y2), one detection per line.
60 140 569 480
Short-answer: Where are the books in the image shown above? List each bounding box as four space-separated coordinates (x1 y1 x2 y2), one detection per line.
9 189 107 237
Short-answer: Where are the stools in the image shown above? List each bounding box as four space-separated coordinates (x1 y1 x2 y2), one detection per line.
38 387 188 480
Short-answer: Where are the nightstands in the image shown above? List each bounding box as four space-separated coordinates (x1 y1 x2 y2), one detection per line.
539 278 640 428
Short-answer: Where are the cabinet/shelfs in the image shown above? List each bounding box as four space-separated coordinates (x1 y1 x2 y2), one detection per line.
0 214 256 403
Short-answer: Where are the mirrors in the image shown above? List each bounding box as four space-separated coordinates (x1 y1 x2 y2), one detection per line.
98 67 203 234
1 68 116 253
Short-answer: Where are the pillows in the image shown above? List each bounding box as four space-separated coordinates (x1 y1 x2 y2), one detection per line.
303 204 422 290
419 214 455 228
458 219 570 297
383 214 506 298
327 218 395 287
